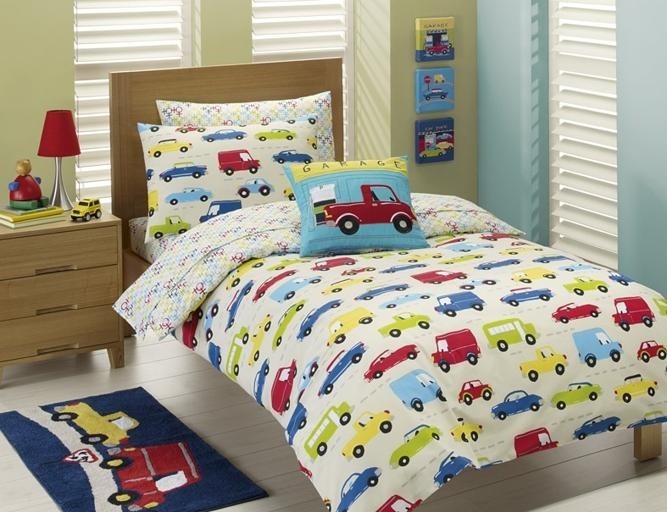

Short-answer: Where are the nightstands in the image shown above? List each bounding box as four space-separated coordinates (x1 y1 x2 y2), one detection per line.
0 202 125 389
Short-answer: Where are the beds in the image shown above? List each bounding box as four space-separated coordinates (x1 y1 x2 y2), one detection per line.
108 59 666 511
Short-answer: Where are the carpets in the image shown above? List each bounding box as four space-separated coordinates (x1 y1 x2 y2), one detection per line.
0 387 269 511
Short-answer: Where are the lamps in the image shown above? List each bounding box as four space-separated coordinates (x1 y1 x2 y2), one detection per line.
36 110 81 212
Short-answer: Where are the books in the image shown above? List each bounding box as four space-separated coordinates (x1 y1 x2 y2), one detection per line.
0 204 67 229
